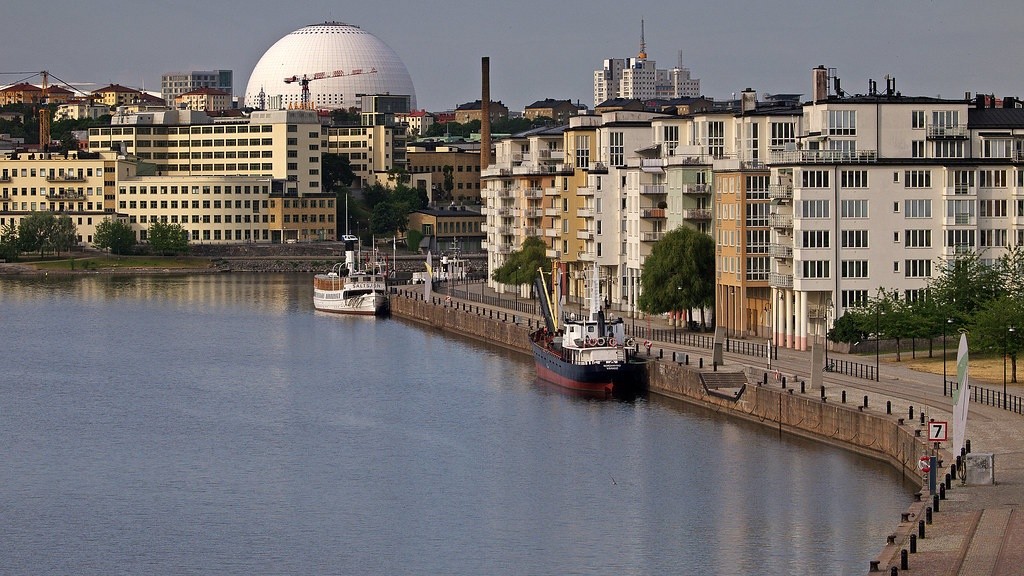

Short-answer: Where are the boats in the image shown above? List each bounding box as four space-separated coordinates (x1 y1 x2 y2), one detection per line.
313 192 387 316
528 257 642 395
365 239 491 286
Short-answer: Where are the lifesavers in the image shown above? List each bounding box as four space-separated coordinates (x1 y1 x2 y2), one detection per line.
644 340 652 349
609 338 617 347
918 456 930 472
589 338 597 346
597 337 605 346
775 369 779 383
626 339 636 347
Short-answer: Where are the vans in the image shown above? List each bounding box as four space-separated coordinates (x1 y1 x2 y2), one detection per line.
342 234 359 243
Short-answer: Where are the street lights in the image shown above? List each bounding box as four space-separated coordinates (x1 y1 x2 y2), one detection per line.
1003 319 1016 410
674 278 682 343
876 303 887 382
726 285 735 352
943 312 953 396
825 297 835 372
515 261 522 311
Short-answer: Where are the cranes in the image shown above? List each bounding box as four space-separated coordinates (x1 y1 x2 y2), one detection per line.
0 69 106 149
284 67 378 111
